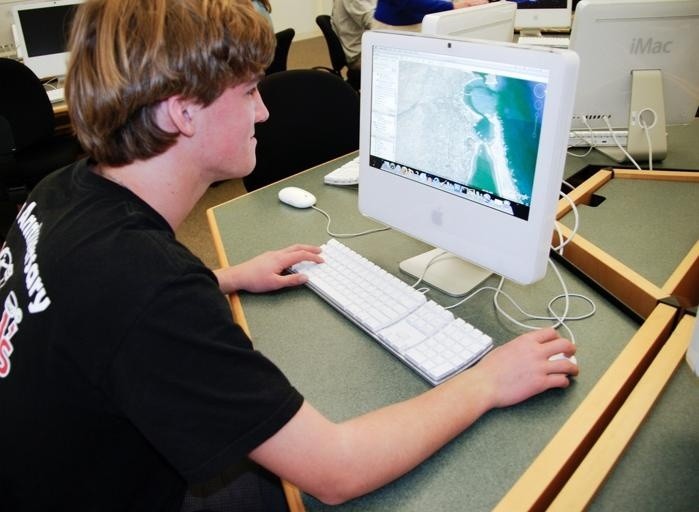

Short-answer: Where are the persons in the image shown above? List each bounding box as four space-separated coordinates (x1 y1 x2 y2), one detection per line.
327 0 382 73
373 0 490 27
1 0 582 512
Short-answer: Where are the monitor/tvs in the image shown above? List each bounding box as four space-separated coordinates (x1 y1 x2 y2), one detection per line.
10 0 89 79
358 30 581 296
422 0 517 43
501 0 572 36
572 0 699 160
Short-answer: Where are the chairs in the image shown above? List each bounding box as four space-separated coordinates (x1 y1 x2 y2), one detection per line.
313 12 344 67
0 55 54 226
234 67 361 194
270 24 295 73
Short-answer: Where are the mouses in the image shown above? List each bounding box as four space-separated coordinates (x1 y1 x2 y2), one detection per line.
278 186 317 208
549 352 577 365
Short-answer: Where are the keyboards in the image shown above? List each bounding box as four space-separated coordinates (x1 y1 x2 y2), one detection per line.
46 87 66 104
284 237 493 385
567 130 669 149
324 156 360 185
519 37 569 48
